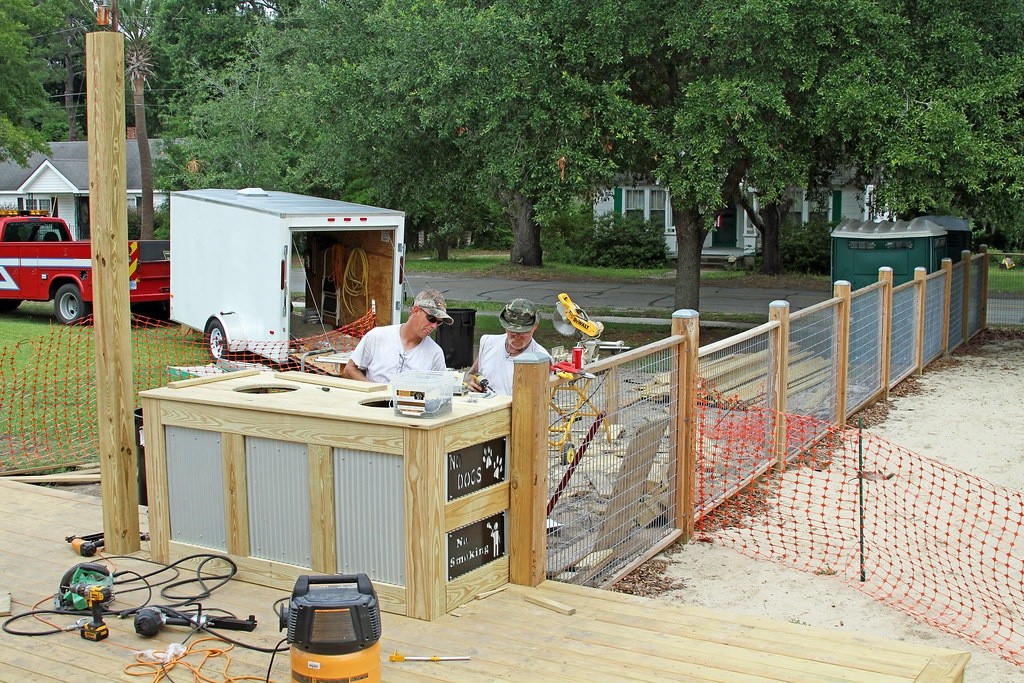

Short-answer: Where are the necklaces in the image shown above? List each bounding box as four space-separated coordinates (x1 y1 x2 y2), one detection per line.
505 337 531 358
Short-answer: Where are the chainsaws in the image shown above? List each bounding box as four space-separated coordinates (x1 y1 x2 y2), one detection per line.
552 292 605 364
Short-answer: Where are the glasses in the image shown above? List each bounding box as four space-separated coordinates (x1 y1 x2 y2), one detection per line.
511 332 530 337
420 307 444 326
396 354 405 373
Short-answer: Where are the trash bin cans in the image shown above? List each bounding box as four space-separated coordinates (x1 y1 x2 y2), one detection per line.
436 308 478 369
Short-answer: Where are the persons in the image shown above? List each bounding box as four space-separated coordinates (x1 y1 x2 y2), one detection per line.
343 289 454 383
464 298 554 396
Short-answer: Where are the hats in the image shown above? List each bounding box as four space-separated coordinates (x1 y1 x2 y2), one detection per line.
414 289 454 325
499 298 538 332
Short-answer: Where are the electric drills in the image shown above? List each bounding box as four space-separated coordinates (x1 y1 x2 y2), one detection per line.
61 582 113 642
133 606 258 638
65 530 151 558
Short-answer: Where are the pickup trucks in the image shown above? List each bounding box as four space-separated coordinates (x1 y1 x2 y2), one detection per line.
0 209 170 326
